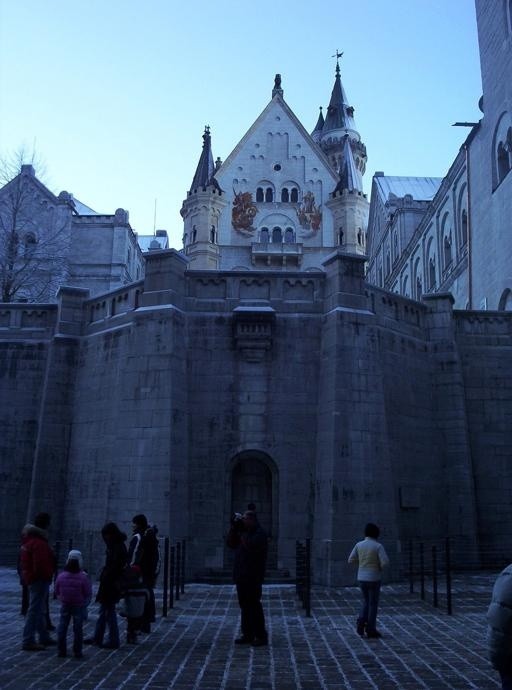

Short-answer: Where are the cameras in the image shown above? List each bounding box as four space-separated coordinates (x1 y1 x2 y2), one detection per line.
232 511 241 520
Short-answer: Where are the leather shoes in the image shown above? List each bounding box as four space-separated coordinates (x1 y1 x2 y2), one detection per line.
367 630 382 638
84 637 96 644
250 638 268 647
99 642 119 649
21 642 45 651
357 619 365 635
41 638 58 644
235 637 255 644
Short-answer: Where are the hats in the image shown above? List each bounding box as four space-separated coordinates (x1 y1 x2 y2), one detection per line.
68 550 83 562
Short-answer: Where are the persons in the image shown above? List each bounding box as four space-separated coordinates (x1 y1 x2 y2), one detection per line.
348 523 389 638
17 512 160 658
486 565 512 690
226 511 269 646
